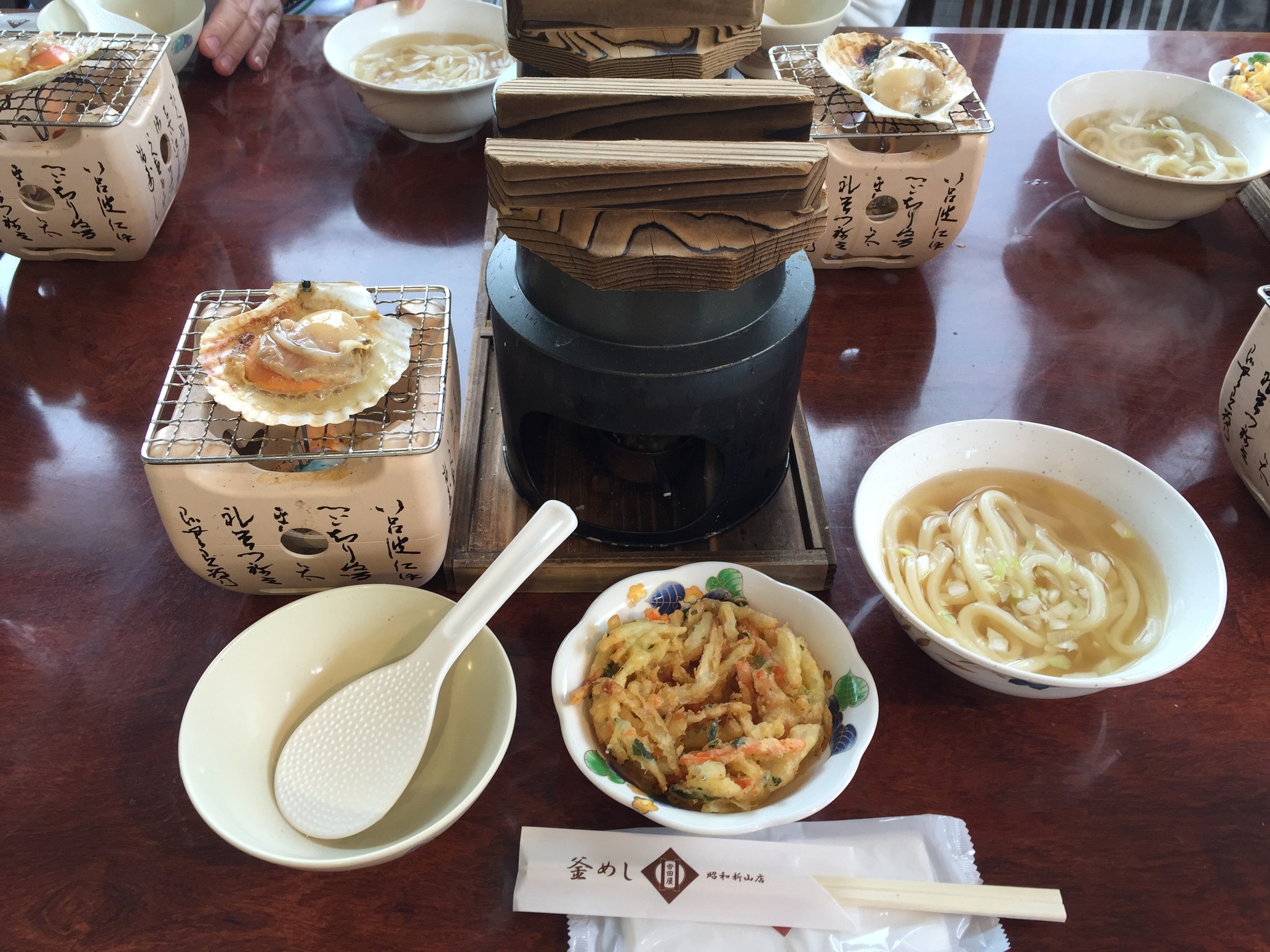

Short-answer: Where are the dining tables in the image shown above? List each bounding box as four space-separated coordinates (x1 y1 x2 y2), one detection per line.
0 13 1270 952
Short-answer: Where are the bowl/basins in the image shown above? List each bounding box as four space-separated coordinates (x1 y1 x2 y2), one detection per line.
853 419 1228 700
736 0 853 79
177 584 519 874
548 560 879 837
323 1 515 144
1048 69 1270 230
36 1 205 85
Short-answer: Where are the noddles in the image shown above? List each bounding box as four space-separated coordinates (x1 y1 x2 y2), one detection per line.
884 468 1170 678
1065 105 1248 181
352 32 507 92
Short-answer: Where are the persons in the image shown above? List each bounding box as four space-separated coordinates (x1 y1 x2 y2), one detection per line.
197 0 285 77
352 0 424 23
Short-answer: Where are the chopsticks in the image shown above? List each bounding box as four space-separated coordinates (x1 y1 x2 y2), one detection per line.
817 872 1068 924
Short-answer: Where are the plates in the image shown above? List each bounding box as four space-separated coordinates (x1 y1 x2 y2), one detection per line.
1206 50 1270 87
